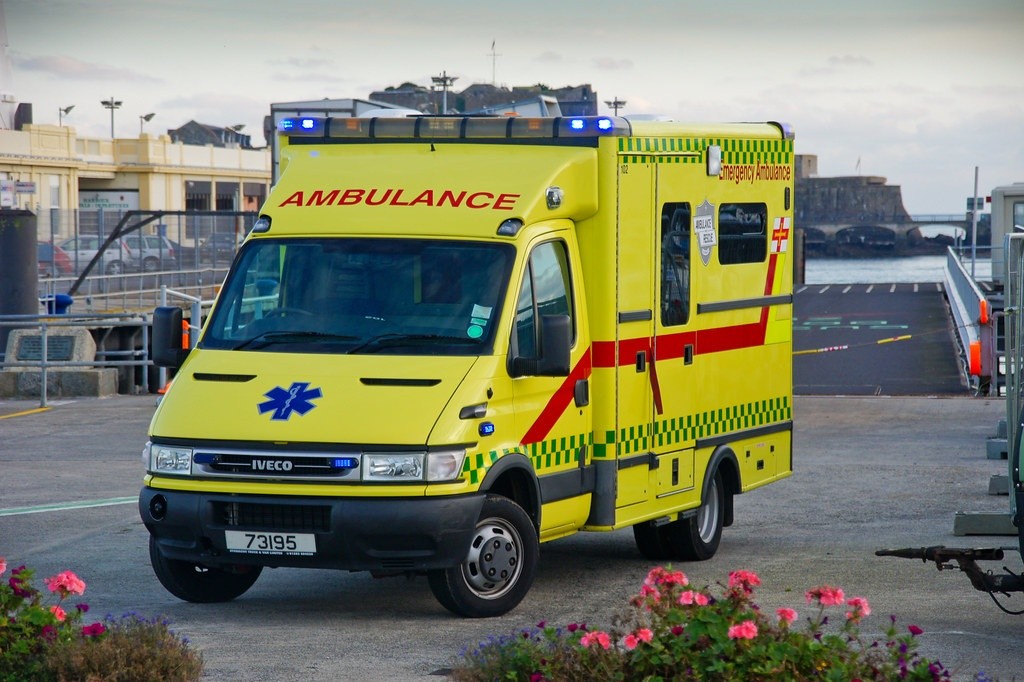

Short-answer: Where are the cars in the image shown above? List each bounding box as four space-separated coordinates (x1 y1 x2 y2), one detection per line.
57 232 135 275
197 230 244 267
36 240 74 280
117 233 179 275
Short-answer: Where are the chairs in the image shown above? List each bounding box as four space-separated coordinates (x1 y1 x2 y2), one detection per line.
306 263 381 313
464 258 512 309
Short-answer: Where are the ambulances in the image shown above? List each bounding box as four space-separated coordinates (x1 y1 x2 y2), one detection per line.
136 113 795 619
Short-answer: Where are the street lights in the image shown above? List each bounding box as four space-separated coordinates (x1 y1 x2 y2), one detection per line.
49 205 61 298
